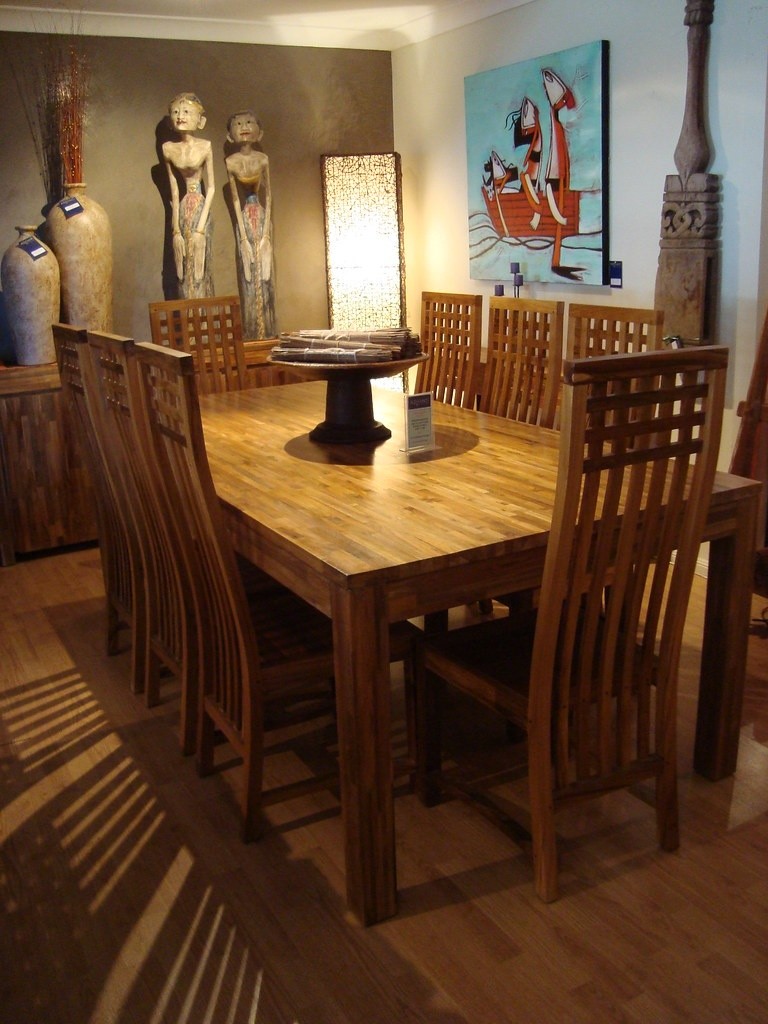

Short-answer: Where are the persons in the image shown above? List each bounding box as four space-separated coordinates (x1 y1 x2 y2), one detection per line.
162 93 222 344
225 112 279 341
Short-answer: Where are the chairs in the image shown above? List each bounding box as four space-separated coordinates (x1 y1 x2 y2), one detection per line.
50 291 729 904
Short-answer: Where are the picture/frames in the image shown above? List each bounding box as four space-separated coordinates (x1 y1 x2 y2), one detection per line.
464 40 609 286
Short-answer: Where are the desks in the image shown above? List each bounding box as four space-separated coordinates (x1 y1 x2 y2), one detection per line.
153 380 762 926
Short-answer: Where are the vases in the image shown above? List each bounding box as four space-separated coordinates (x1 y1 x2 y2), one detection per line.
0 222 60 366
46 184 114 333
